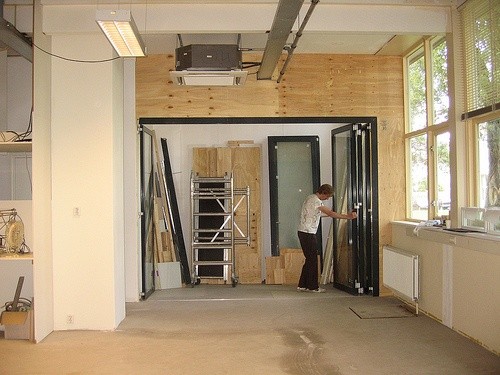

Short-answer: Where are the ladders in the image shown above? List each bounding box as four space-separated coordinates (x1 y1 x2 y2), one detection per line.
189 169 250 287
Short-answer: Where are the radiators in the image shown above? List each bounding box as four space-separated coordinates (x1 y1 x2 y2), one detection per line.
382 243 419 303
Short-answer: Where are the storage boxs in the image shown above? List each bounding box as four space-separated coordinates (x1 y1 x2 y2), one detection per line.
0 311 31 340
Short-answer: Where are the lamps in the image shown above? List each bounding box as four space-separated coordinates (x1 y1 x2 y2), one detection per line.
94 1 148 58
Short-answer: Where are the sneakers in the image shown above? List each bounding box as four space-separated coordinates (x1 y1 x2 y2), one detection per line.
308 287 327 293
297 287 310 292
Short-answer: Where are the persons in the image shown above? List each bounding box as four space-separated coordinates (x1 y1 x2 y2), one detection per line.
295 184 358 293
